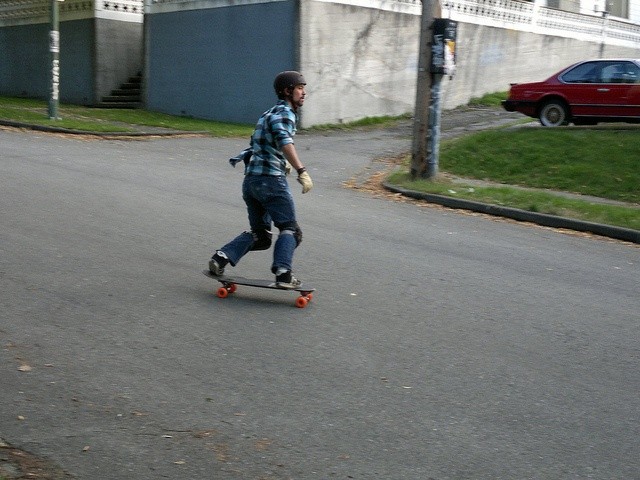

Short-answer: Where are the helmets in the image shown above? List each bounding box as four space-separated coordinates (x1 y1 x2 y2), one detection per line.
275 71 307 90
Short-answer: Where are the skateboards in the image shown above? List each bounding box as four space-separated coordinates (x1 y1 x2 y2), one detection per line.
203 269 316 308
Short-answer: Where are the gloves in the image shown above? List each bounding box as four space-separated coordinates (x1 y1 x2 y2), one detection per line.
297 170 312 193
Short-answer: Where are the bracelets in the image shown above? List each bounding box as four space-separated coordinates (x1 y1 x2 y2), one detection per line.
297 167 306 174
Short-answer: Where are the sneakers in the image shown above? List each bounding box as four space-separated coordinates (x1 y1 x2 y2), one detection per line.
208 253 226 276
277 271 303 289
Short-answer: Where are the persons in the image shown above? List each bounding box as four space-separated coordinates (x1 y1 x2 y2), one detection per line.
209 70 313 290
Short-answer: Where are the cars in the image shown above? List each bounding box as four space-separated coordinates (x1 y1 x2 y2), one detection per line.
501 58 640 127
